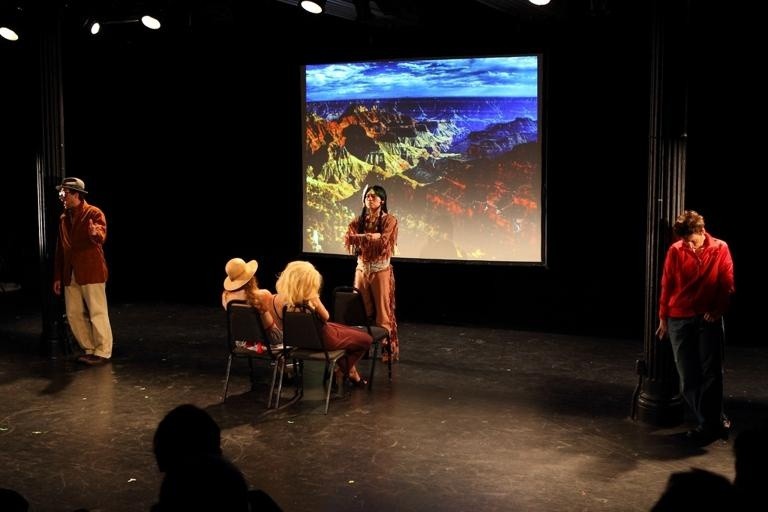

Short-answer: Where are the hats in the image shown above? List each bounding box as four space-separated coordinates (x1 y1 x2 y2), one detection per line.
223 257 258 291
56 177 89 194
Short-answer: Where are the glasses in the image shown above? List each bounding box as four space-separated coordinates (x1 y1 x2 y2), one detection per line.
59 191 71 197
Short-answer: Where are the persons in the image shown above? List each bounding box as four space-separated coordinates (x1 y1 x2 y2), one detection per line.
266 259 371 388
344 184 399 361
644 417 767 512
219 257 300 385
654 210 737 444
151 403 225 511
52 176 112 367
185 459 283 512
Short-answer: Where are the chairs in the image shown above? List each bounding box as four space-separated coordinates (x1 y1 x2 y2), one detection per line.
220 286 392 415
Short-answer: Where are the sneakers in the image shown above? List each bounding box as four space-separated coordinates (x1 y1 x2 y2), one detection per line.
78 354 111 365
686 413 731 442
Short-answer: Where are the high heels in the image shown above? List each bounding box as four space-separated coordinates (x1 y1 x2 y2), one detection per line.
330 370 368 389
